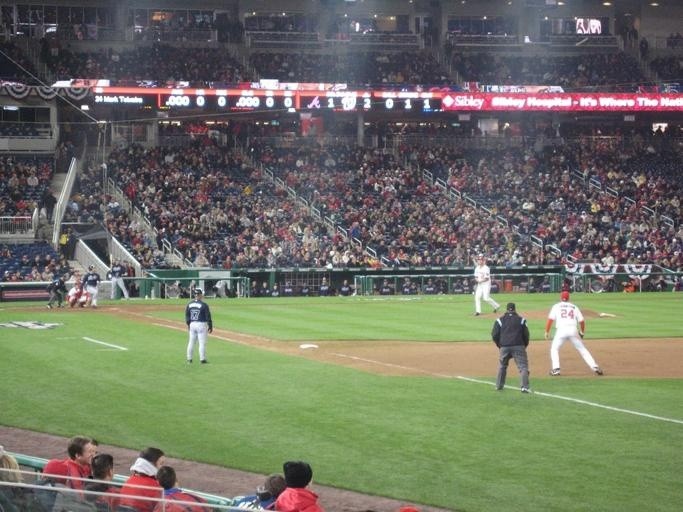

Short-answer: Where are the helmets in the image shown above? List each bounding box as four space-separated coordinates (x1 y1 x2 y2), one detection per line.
88 265 95 271
474 253 486 265
112 258 120 266
63 273 70 280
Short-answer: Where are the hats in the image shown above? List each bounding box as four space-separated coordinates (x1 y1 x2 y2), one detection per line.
507 303 515 311
264 473 288 498
41 459 68 483
283 461 312 488
194 289 202 294
561 291 570 301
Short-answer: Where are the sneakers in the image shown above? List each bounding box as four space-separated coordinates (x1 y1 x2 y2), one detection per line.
473 312 480 316
521 386 532 393
549 368 561 376
46 302 99 310
493 306 500 313
593 367 602 376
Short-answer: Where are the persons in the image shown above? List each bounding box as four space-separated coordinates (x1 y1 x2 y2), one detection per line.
0 434 327 512
544 291 604 378
2 107 681 309
185 287 216 363
2 1 682 93
490 302 532 395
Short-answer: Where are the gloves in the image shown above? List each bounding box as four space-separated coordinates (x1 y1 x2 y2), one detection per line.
579 330 584 339
